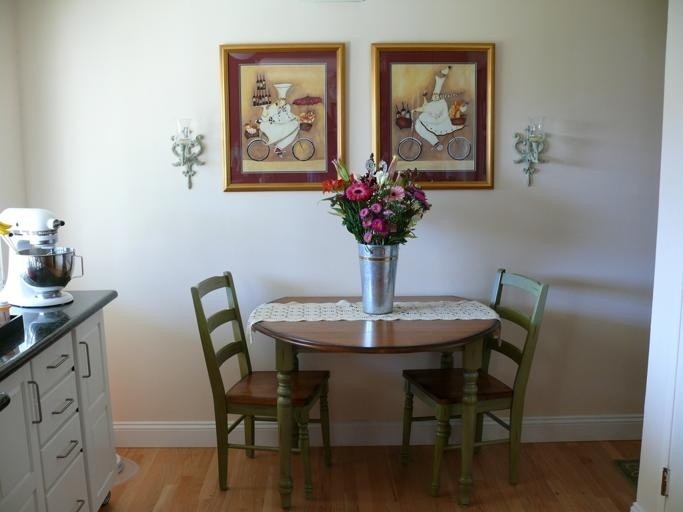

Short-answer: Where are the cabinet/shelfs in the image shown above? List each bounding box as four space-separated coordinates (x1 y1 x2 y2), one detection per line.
0 310 119 511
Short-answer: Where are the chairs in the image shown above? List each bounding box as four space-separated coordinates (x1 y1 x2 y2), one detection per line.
401 268 548 506
189 271 330 503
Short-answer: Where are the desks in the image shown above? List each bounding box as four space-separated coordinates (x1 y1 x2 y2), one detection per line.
252 295 502 511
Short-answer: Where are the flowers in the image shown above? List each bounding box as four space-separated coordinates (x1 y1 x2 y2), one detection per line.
325 154 432 244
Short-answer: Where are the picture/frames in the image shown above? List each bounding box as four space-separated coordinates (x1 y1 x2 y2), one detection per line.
219 41 348 192
371 42 494 192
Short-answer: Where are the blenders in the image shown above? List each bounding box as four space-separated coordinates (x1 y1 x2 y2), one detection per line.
1 208 83 307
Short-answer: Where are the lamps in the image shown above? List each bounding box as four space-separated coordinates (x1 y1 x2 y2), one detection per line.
169 110 206 191
513 115 549 185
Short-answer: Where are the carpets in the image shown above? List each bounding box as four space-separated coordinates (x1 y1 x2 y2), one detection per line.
613 458 639 492
